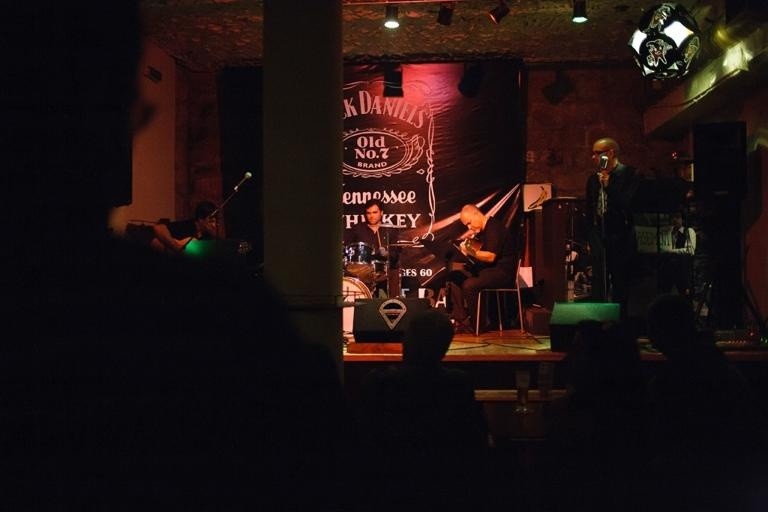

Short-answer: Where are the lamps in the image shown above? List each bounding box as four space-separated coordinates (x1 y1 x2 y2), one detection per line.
382 1 589 33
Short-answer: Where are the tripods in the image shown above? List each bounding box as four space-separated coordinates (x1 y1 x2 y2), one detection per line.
696 204 768 336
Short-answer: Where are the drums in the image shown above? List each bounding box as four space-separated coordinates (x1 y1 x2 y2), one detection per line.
344 242 385 275
342 276 372 334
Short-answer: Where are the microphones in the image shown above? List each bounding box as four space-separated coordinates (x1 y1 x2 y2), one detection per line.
234 172 252 192
599 155 609 170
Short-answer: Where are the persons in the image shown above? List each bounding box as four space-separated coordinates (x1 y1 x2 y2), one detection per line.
1 1 361 510
362 313 496 510
579 137 637 318
449 204 516 334
348 196 399 296
571 336 646 512
154 200 220 264
646 295 767 512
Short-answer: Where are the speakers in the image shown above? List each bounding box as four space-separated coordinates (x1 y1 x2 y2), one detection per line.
548 301 624 352
692 120 748 204
353 297 430 342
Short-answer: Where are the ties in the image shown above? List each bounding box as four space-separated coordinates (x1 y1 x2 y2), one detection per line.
596 179 608 219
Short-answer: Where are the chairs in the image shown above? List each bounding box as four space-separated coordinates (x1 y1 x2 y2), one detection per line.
474 256 524 334
